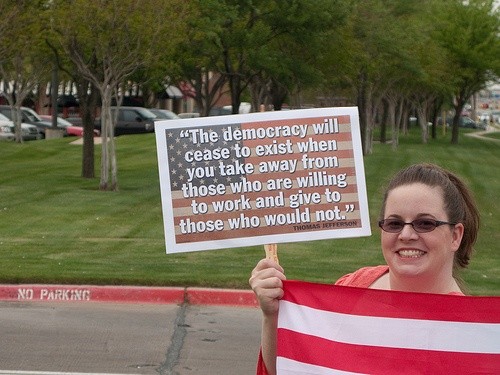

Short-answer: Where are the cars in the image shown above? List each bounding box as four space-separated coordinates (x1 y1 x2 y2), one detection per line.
410 116 478 129
0 102 317 143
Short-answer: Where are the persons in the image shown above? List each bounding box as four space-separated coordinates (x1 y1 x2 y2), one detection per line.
249 163 478 375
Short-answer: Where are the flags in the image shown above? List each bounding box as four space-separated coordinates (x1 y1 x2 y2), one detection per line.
276 279 500 375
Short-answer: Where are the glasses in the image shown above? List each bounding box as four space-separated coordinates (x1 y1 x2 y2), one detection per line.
379 219 456 233
